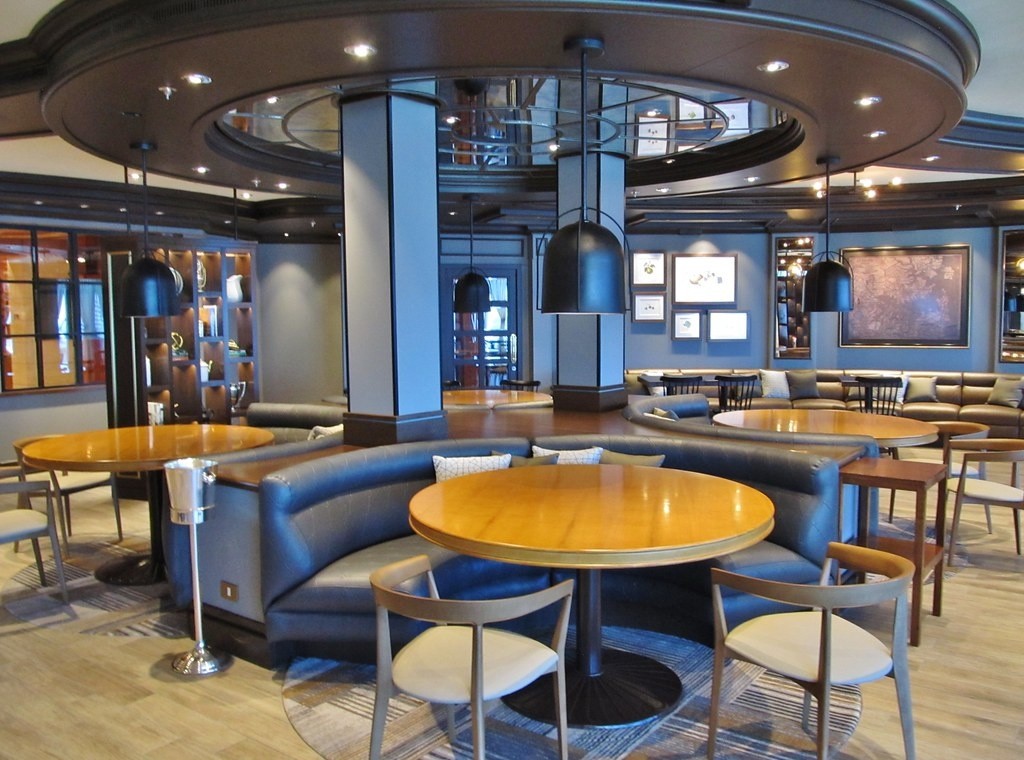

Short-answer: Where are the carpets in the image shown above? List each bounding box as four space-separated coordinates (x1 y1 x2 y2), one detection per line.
280 606 864 760
0 534 193 638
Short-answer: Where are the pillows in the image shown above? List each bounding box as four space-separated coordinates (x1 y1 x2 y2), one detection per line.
904 376 939 402
593 445 666 468
879 374 907 405
532 445 603 464
760 369 790 399
845 374 881 401
433 454 511 482
785 371 820 400
732 374 763 401
641 372 667 396
491 449 559 468
986 377 1024 408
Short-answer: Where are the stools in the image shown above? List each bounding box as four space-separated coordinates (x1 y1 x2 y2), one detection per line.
500 380 541 392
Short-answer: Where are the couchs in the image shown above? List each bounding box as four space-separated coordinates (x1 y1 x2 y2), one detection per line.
165 367 1024 675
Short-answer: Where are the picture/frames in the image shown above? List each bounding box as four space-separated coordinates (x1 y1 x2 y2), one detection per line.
707 309 751 342
629 249 667 287
671 309 702 341
632 291 667 322
672 253 738 305
837 245 973 350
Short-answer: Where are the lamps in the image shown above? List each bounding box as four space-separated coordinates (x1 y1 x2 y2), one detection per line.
536 38 633 316
119 141 179 317
451 193 491 312
802 156 855 313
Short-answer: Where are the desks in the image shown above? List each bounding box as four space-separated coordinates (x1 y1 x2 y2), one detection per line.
408 464 775 728
21 423 274 587
837 456 948 645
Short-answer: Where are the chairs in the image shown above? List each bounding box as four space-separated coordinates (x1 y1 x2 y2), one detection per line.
713 374 758 414
945 439 1024 568
658 376 702 397
710 543 916 760
369 553 575 760
888 420 990 523
848 377 903 415
0 481 69 602
12 435 123 554
